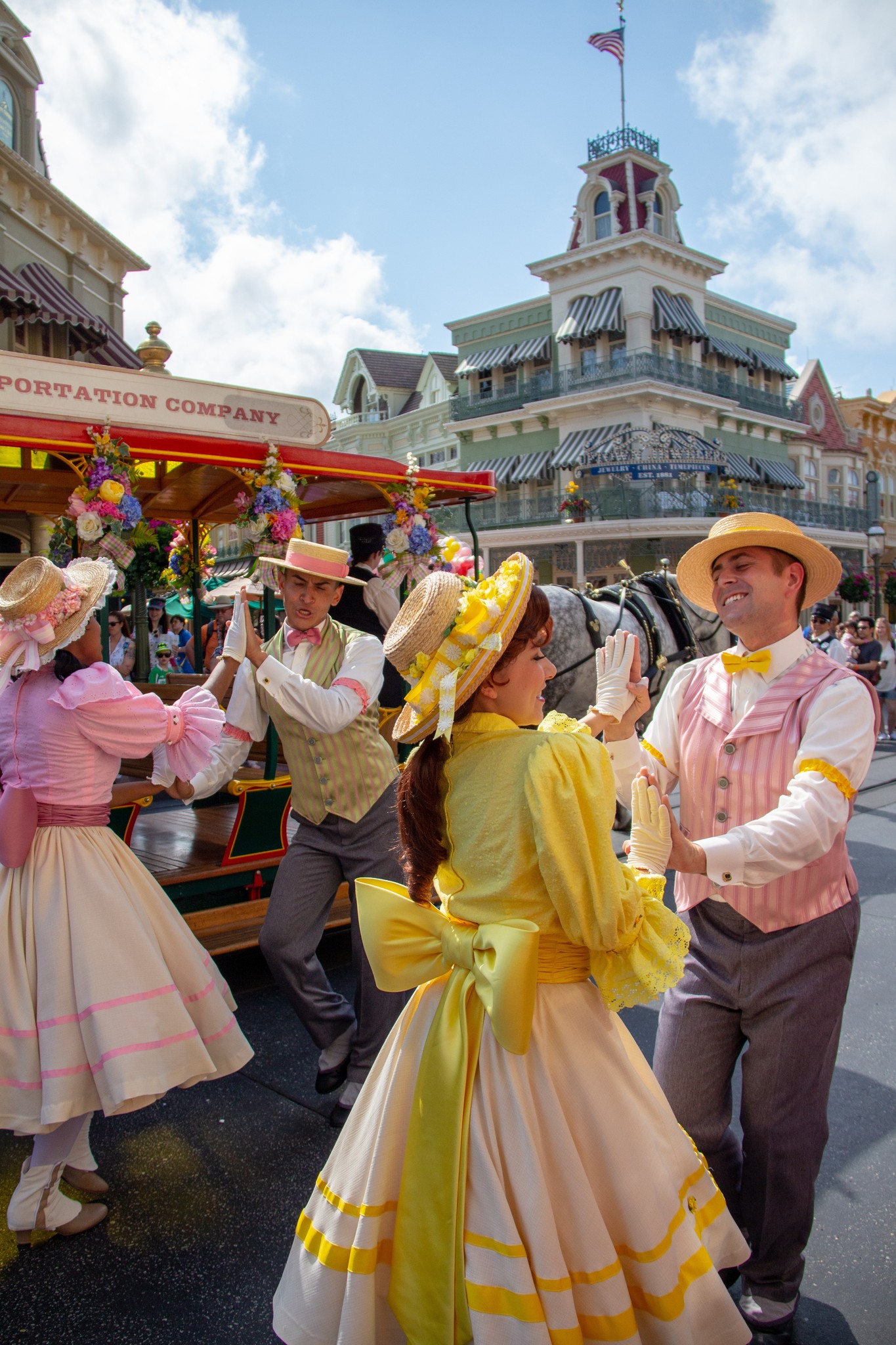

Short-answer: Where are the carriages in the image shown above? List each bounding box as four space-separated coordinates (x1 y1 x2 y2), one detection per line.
2 347 741 966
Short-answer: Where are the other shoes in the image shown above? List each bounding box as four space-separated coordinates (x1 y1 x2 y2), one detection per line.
314 1053 355 1127
878 732 887 740
887 733 896 740
716 1266 800 1345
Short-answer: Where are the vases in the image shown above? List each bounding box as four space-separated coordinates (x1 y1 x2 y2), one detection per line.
573 516 585 523
718 511 727 518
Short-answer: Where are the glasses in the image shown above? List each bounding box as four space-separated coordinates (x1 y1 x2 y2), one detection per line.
858 625 870 630
153 630 159 639
157 652 171 658
215 608 231 613
109 621 120 627
811 619 827 625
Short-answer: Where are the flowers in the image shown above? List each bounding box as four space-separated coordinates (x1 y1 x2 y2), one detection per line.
159 523 217 587
0 583 91 639
410 560 521 681
384 452 440 553
559 481 591 516
234 441 308 542
62 416 143 540
712 479 745 509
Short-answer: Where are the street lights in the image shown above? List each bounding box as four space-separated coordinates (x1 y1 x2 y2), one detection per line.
864 519 887 621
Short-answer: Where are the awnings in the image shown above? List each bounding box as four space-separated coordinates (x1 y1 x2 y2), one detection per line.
555 289 626 347
453 335 551 381
547 422 632 471
703 335 799 382
653 422 722 461
651 288 709 346
720 451 805 491
465 450 554 486
0 262 144 371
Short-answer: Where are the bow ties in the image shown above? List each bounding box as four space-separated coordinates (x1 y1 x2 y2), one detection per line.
720 649 773 675
287 627 322 647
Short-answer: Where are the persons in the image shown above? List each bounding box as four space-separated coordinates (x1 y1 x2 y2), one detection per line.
164 538 405 1130
328 520 411 709
273 551 760 1345
0 557 254 1249
603 512 880 1323
802 602 896 740
107 595 281 685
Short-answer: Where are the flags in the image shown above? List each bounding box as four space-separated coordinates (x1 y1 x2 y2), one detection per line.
591 25 622 65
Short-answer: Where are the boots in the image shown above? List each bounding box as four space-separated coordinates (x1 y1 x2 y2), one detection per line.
7 1155 107 1245
31 1109 109 1196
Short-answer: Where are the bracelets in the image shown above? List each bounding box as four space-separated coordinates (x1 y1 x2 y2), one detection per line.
855 664 858 670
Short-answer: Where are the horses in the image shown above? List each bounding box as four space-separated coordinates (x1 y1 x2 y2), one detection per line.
519 567 737 836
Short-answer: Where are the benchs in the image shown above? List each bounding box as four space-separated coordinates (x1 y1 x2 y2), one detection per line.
106 681 202 804
166 670 294 791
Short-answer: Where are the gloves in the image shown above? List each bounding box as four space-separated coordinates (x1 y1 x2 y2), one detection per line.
145 743 177 788
625 776 672 878
215 591 247 665
585 628 649 724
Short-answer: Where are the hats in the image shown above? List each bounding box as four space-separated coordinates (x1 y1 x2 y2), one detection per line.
381 550 535 744
259 538 369 586
154 641 173 655
811 602 836 623
147 598 166 609
349 523 387 551
207 595 234 609
0 555 118 695
676 511 843 615
